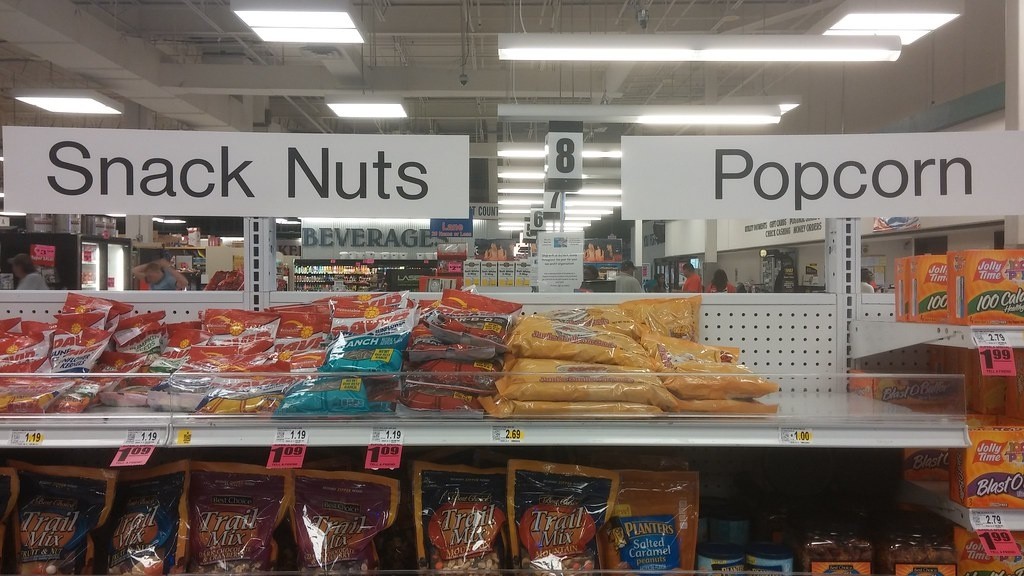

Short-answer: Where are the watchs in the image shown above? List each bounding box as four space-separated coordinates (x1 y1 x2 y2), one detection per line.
167 264 175 272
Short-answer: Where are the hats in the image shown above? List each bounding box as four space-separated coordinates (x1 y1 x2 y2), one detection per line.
621 261 637 270
8 253 32 268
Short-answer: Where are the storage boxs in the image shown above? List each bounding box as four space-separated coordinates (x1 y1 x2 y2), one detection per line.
463 260 533 287
848 244 1024 576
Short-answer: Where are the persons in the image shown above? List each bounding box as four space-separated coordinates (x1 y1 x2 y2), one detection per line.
12 253 51 290
644 273 668 293
861 268 876 293
609 261 645 293
671 263 701 293
130 257 188 290
705 269 736 293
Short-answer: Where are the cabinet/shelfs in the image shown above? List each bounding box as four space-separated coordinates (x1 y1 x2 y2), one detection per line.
846 217 1023 576
1 205 971 576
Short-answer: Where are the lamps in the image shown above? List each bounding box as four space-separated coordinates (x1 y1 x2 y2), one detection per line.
498 141 621 238
231 0 367 44
498 33 903 61
325 92 410 119
11 86 124 116
498 104 781 123
